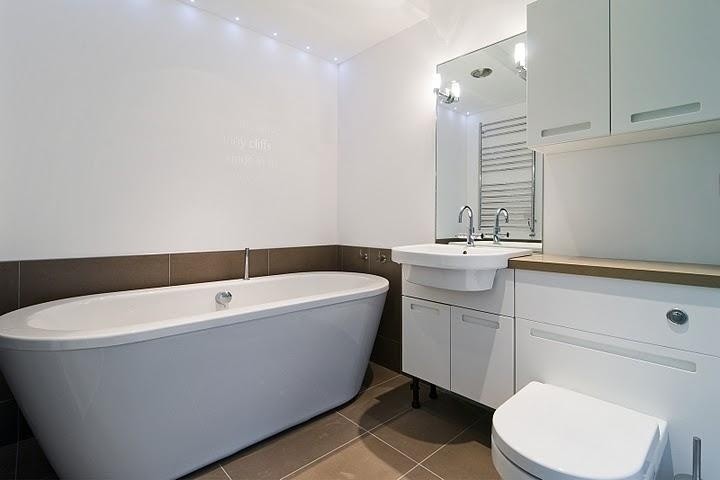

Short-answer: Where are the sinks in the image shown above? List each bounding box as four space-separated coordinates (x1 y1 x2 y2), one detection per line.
391 244 532 291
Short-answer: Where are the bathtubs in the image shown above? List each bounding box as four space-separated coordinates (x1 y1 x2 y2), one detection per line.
0 270 390 480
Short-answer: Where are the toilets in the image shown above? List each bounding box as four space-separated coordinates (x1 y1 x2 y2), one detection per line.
491 380 669 480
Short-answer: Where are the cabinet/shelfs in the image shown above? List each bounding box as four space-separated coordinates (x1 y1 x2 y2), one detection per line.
527 0 720 149
401 269 515 412
514 271 720 480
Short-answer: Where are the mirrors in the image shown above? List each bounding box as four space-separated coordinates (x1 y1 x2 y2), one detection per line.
431 28 544 244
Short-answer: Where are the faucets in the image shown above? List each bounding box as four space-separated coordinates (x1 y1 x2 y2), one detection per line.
495 208 509 227
458 205 473 243
